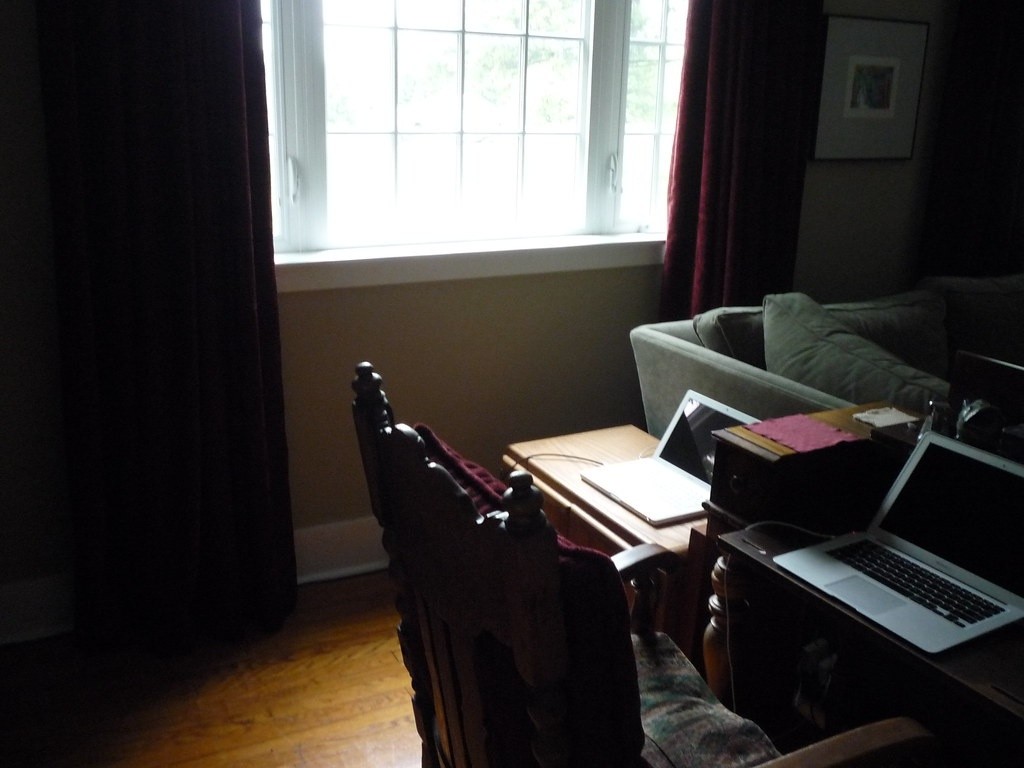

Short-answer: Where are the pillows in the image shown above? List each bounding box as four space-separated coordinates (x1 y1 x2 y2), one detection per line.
761 275 1024 432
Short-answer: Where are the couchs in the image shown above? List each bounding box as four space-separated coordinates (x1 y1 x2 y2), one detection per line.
628 294 1024 435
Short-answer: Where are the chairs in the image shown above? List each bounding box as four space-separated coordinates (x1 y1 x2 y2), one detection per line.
351 361 932 768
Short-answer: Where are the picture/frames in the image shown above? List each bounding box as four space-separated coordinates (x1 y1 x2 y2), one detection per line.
811 14 930 162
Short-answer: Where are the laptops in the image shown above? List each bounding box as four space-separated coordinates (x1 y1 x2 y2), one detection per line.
580 389 763 526
773 432 1024 653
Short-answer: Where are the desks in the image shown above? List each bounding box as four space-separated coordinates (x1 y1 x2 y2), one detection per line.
703 498 1024 768
503 423 706 635
